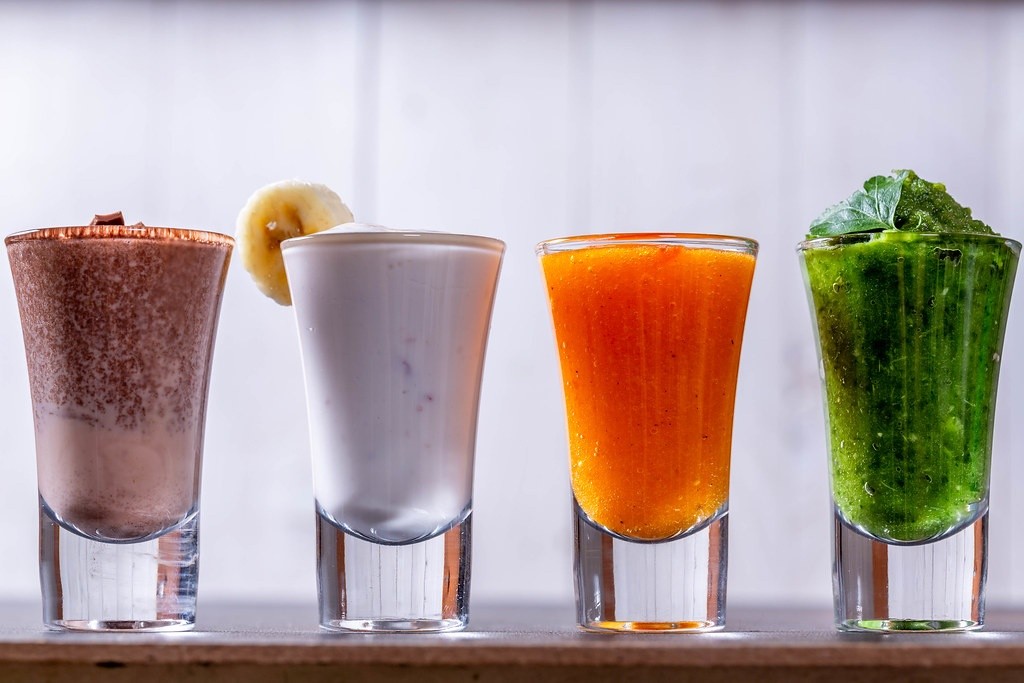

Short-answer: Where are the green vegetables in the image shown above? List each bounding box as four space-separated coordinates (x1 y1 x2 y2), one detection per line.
809 176 921 232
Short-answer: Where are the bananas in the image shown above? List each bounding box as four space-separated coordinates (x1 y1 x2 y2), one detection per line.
236 181 354 307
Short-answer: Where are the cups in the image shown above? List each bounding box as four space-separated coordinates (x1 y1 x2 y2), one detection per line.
796 232 1023 633
4 225 235 633
534 233 759 634
280 230 507 632
